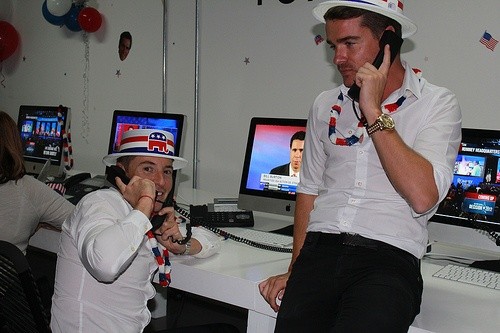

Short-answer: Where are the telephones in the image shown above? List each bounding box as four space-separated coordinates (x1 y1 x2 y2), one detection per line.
62 173 112 196
348 30 404 104
106 165 157 203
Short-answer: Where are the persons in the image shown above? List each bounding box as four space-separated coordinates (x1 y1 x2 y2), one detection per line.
128 125 134 131
50 128 221 332
258 0 463 333
0 110 77 294
264 131 306 194
449 159 500 210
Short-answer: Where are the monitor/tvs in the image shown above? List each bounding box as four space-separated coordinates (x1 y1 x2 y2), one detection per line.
427 128 500 232
16 105 71 177
236 117 307 216
104 110 186 200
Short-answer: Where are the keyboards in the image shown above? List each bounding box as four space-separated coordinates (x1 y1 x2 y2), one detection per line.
236 229 295 249
432 263 500 292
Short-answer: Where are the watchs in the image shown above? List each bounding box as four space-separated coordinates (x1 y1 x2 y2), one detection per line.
366 113 395 135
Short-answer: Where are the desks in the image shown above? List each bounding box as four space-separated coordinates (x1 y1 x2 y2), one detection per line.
29 192 500 332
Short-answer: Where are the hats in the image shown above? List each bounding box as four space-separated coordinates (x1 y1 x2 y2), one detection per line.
310 0 418 39
102 129 188 172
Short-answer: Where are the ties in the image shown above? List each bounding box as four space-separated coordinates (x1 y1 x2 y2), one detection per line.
289 174 297 194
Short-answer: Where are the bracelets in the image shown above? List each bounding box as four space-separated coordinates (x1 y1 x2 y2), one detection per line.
175 236 191 255
